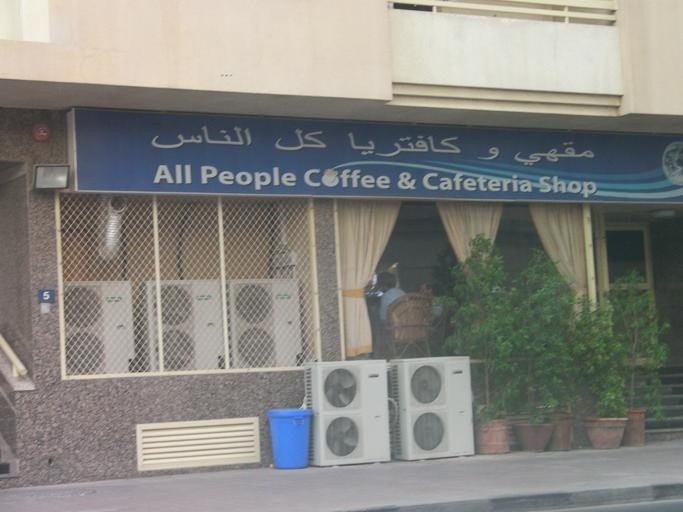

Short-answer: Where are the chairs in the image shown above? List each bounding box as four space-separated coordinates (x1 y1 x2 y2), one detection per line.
383 288 437 359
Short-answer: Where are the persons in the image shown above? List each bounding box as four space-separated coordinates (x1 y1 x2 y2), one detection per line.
420 281 441 315
375 272 408 328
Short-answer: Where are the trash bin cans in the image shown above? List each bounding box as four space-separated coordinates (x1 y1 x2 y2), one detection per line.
267 410 312 469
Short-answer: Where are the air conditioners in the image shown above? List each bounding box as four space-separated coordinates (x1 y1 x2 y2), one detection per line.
146 276 226 373
304 360 394 466
229 277 303 368
62 280 137 375
398 352 475 462
